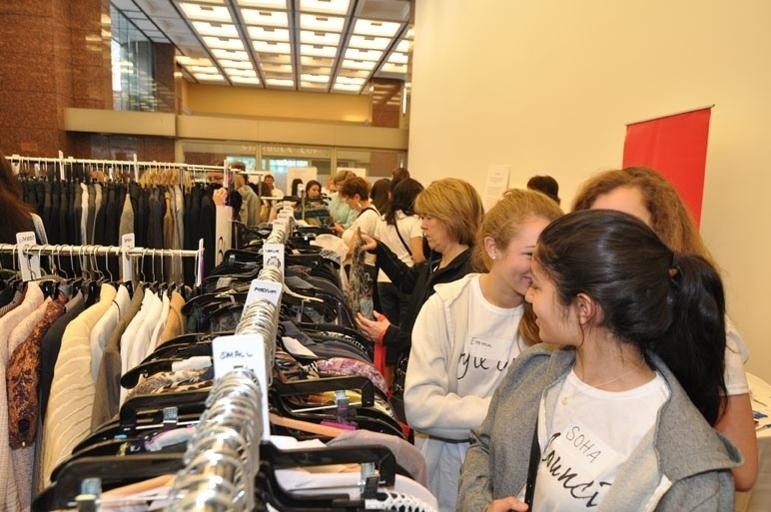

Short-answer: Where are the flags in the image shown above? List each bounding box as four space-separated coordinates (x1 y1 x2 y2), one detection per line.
623 107 709 232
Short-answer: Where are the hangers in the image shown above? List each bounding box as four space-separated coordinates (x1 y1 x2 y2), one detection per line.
3 158 236 213
32 192 393 511
2 240 203 345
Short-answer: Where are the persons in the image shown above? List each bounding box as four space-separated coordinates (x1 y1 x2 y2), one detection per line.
526 175 559 209
210 156 489 416
0 150 48 247
456 208 746 512
568 165 756 492
402 190 565 512
